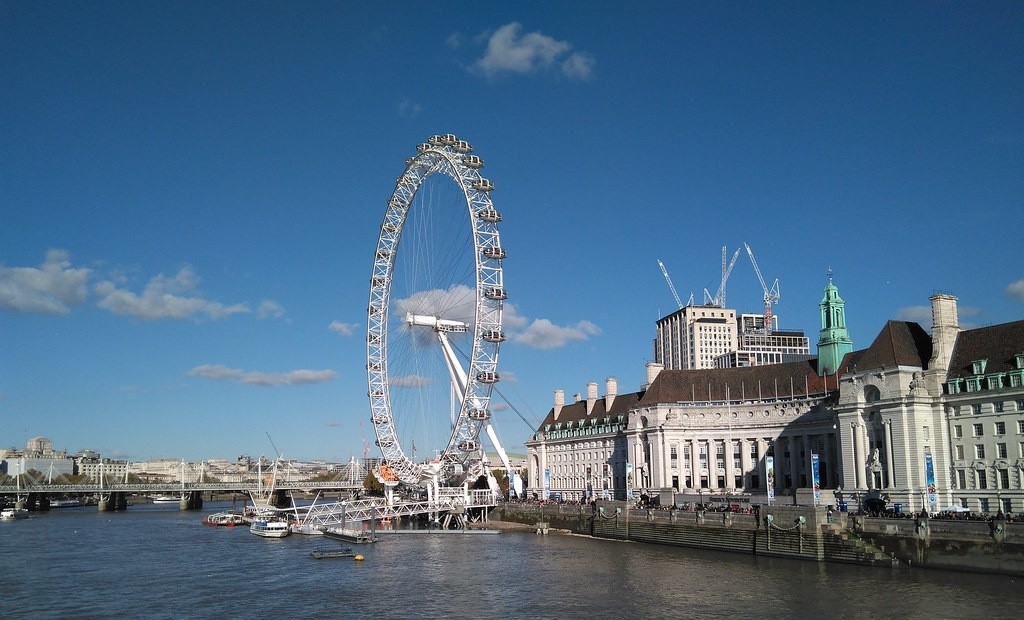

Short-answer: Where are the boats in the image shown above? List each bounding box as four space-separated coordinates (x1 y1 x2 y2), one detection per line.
290 523 324 534
309 543 355 558
250 525 289 538
0 507 30 520
208 512 222 523
354 555 365 561
50 500 80 508
243 505 277 525
212 514 244 527
153 495 189 504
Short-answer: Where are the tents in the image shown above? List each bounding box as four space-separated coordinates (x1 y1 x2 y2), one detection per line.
943 503 970 513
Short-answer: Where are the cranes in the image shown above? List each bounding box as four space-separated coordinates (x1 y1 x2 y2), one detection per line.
657 258 694 309
743 242 780 334
703 244 725 309
714 248 741 305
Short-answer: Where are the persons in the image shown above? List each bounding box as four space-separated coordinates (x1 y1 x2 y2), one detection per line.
497 497 1024 521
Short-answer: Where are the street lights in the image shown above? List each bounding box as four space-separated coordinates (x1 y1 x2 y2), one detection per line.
996 490 1005 520
856 490 865 516
920 490 928 518
650 490 654 509
671 489 678 509
699 489 703 512
